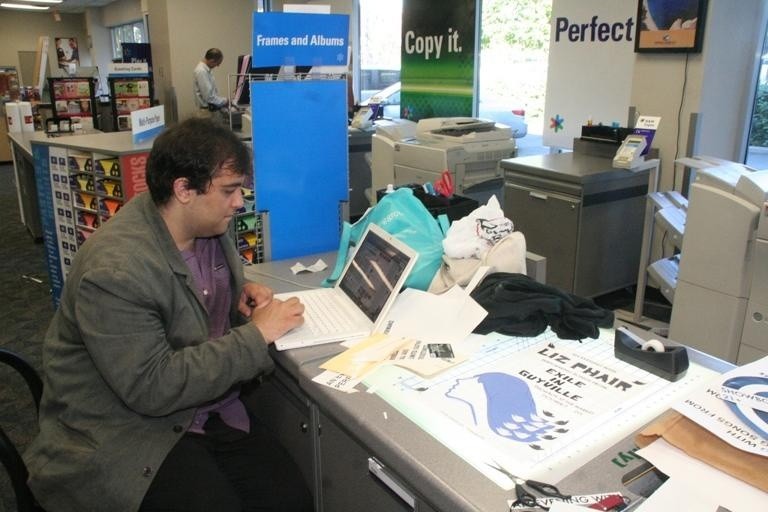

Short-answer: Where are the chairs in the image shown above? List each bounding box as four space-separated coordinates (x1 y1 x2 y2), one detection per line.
0 349 49 512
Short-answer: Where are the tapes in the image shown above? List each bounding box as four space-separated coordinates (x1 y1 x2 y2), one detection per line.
618 326 665 353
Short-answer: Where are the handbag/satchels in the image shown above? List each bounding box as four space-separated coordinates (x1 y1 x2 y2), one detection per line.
207 101 219 112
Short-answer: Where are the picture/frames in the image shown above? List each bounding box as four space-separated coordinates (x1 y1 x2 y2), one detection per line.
633 0 709 54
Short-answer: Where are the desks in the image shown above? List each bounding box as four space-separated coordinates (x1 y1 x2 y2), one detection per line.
241 241 768 512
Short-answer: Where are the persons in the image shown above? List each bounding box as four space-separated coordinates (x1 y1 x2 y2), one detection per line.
61 38 80 69
193 47 230 129
55 38 69 69
21 115 316 511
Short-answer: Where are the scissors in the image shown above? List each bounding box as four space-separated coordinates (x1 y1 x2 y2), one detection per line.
486 461 572 511
434 171 453 199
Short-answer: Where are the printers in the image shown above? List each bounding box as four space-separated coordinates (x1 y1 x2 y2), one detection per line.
371 114 520 216
645 152 768 367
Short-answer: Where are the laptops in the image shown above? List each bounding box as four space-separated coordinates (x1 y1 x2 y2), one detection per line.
264 222 419 353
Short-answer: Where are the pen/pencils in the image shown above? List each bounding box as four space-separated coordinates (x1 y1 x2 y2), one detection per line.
423 182 435 196
